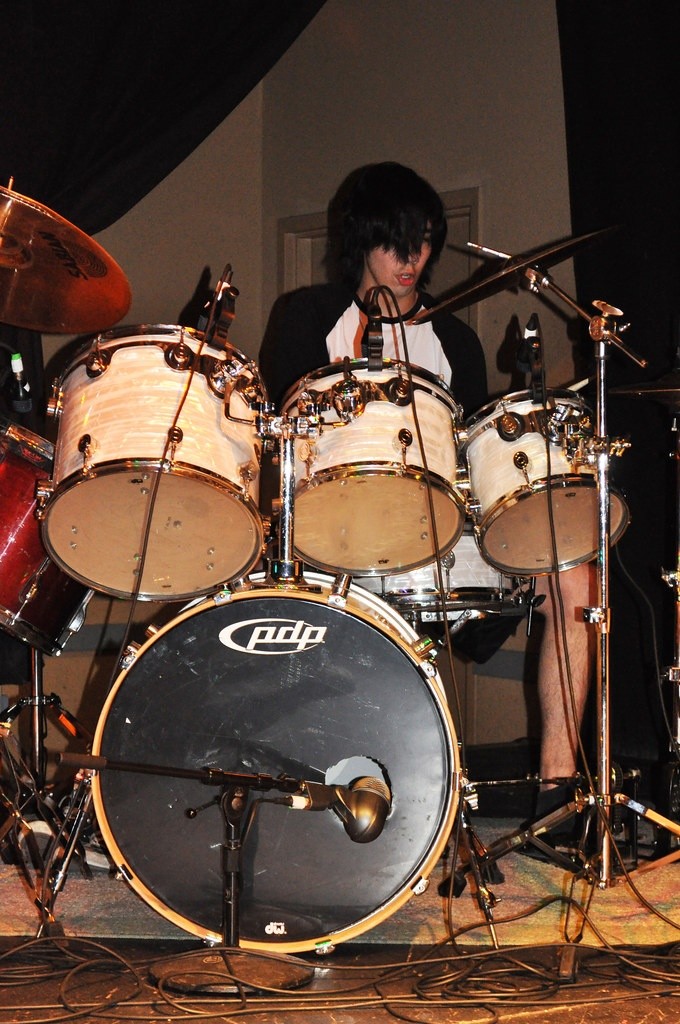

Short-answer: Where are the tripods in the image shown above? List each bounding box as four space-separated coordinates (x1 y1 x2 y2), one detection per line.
1 648 95 881
439 262 680 984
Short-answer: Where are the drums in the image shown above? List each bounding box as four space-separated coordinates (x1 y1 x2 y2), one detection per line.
89 570 461 951
467 387 630 574
41 323 269 603
0 417 97 657
281 355 471 579
346 478 529 627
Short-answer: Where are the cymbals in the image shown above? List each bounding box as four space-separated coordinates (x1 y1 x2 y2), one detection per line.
0 184 132 335
407 231 622 326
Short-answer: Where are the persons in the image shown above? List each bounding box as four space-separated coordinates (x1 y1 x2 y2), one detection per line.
256 160 633 852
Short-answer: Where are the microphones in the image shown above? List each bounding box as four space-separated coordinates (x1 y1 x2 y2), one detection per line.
361 291 382 360
197 265 233 331
521 311 541 365
274 776 392 844
10 352 33 412
